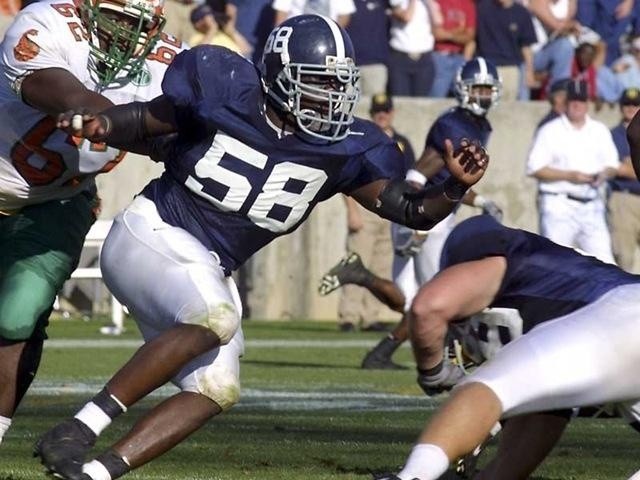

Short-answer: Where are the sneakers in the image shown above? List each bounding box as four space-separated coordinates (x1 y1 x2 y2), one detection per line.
317 251 364 297
360 353 410 371
30 417 98 480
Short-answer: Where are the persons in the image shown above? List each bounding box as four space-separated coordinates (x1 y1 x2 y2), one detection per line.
0 0 640 480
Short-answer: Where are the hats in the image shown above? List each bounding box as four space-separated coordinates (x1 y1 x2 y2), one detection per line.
549 77 589 100
618 87 640 106
369 93 393 114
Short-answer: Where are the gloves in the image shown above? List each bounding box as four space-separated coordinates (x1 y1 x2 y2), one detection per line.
480 200 503 223
417 359 466 399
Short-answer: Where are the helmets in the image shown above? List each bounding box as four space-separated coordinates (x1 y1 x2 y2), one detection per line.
453 57 503 117
79 0 168 87
257 13 363 142
